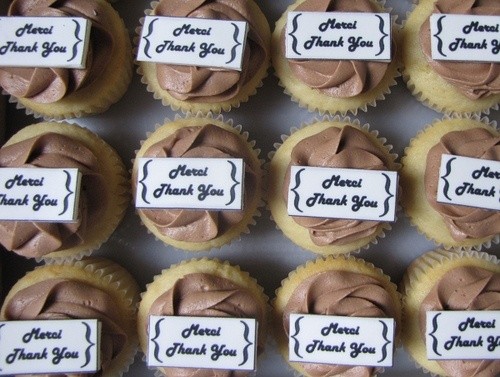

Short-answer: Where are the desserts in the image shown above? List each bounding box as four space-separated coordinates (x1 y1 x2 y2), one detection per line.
0 0 500 377
